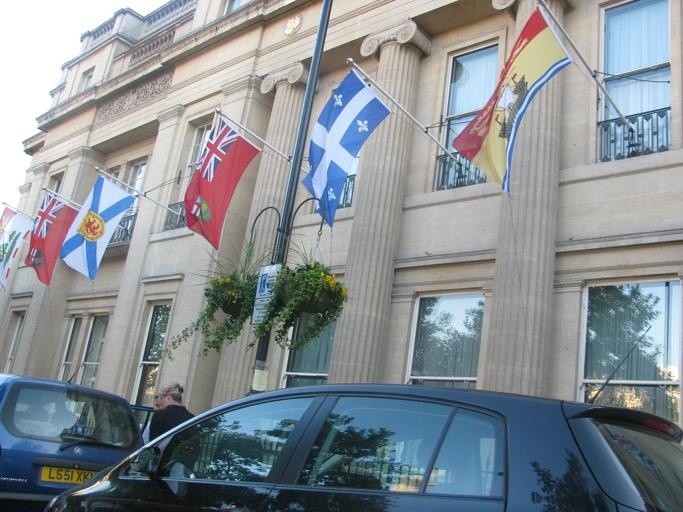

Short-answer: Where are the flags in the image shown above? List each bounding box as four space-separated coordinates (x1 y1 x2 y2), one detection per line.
60 173 136 280
185 116 261 250
302 68 392 226
452 4 572 192
25 191 79 286
0 205 35 288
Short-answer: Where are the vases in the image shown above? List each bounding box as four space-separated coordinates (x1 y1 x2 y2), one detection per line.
280 284 343 316
220 297 251 317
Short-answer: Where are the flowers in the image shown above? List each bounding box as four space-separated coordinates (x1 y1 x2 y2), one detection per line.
246 261 351 354
156 247 272 359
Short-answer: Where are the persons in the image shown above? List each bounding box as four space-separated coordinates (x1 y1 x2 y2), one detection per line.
149 384 197 443
142 388 167 445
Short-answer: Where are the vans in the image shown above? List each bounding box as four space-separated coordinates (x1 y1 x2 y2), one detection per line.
0 347 156 510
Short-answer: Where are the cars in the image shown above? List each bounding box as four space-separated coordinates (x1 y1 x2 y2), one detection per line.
42 324 683 511
